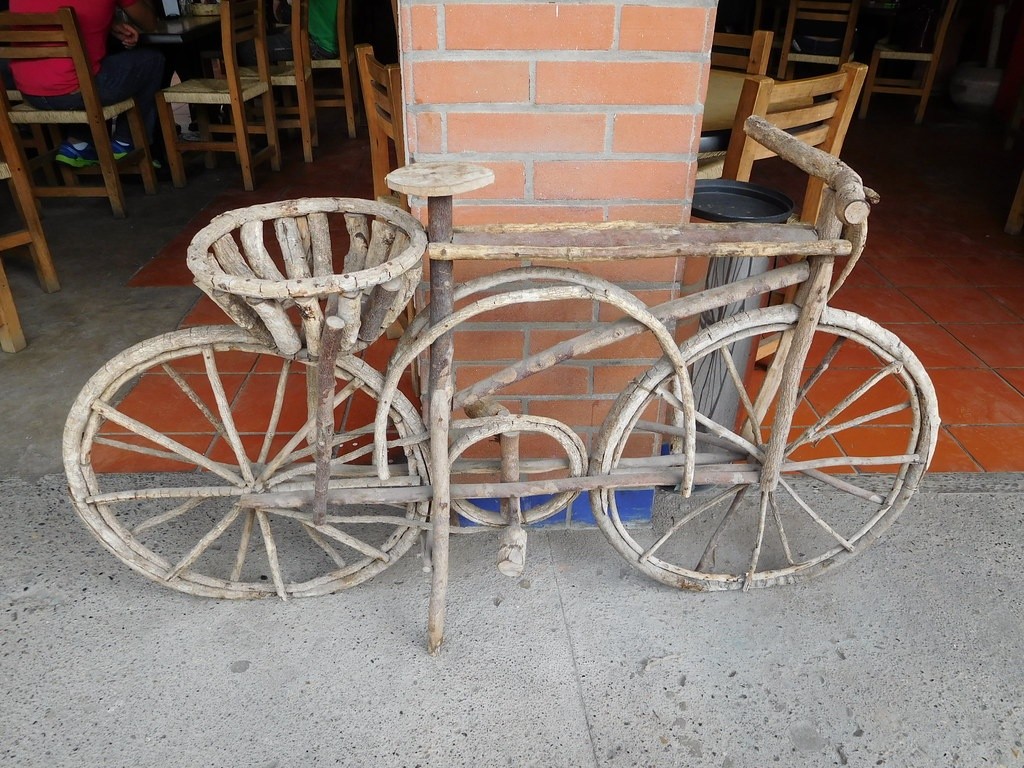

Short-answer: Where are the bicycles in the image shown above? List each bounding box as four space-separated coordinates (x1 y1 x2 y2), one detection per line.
62 114 943 602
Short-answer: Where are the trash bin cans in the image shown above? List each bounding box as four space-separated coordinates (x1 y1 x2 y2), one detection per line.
661 178 798 495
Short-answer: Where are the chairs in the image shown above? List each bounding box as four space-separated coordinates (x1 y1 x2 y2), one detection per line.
1 0 416 354
690 0 1024 363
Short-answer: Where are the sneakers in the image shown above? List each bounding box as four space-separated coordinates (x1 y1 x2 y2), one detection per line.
55 141 99 167
109 140 162 169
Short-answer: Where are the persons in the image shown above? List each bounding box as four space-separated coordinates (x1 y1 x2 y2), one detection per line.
873 0 929 88
793 0 858 74
236 0 337 155
8 0 221 167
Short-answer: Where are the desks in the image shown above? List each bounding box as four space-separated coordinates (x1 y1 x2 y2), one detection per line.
699 69 812 138
110 14 225 80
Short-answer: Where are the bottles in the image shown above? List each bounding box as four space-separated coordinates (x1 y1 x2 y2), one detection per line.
179 0 190 17
